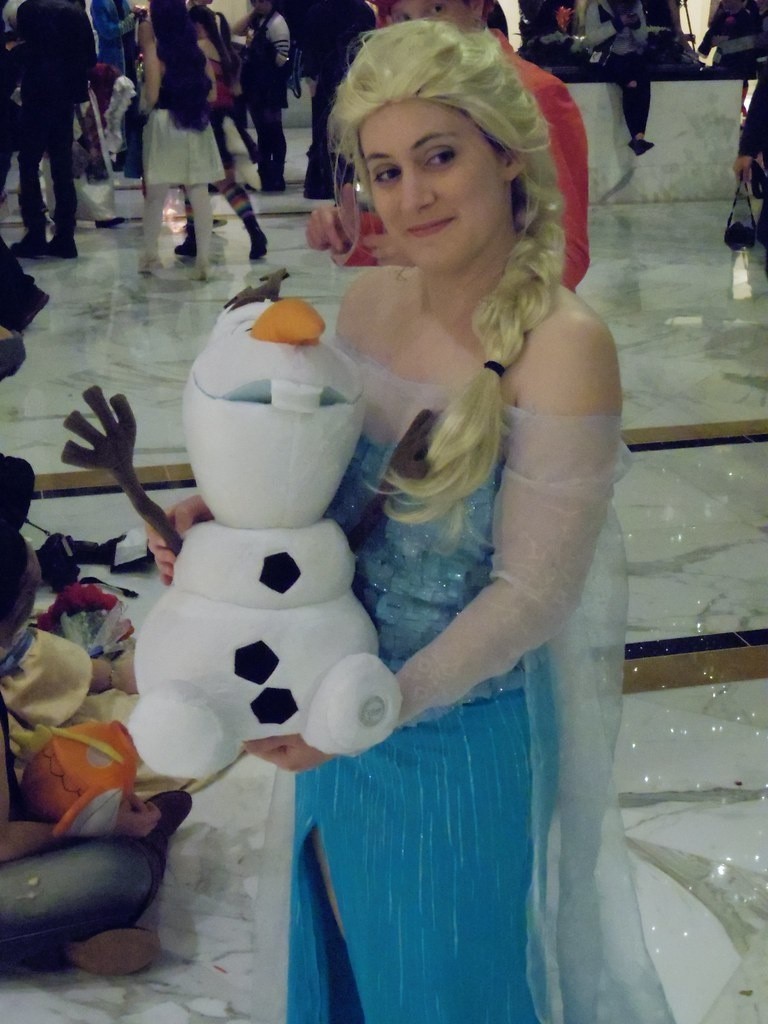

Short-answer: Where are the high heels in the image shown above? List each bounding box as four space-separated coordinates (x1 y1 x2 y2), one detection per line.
186 262 213 283
135 254 158 278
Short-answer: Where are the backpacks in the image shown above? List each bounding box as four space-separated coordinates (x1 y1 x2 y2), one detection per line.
276 39 309 100
11 2 97 110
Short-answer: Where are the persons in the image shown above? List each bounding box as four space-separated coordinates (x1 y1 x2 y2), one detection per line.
0 449 195 978
1 0 767 382
146 13 675 1024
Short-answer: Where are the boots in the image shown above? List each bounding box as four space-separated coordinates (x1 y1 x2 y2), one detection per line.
11 190 48 258
47 184 82 258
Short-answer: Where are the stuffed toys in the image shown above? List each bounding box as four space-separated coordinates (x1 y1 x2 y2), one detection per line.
127 266 406 780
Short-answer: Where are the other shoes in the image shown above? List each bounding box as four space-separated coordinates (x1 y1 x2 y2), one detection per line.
132 789 195 859
247 227 267 258
626 138 654 154
97 215 127 227
22 918 161 976
175 239 200 258
